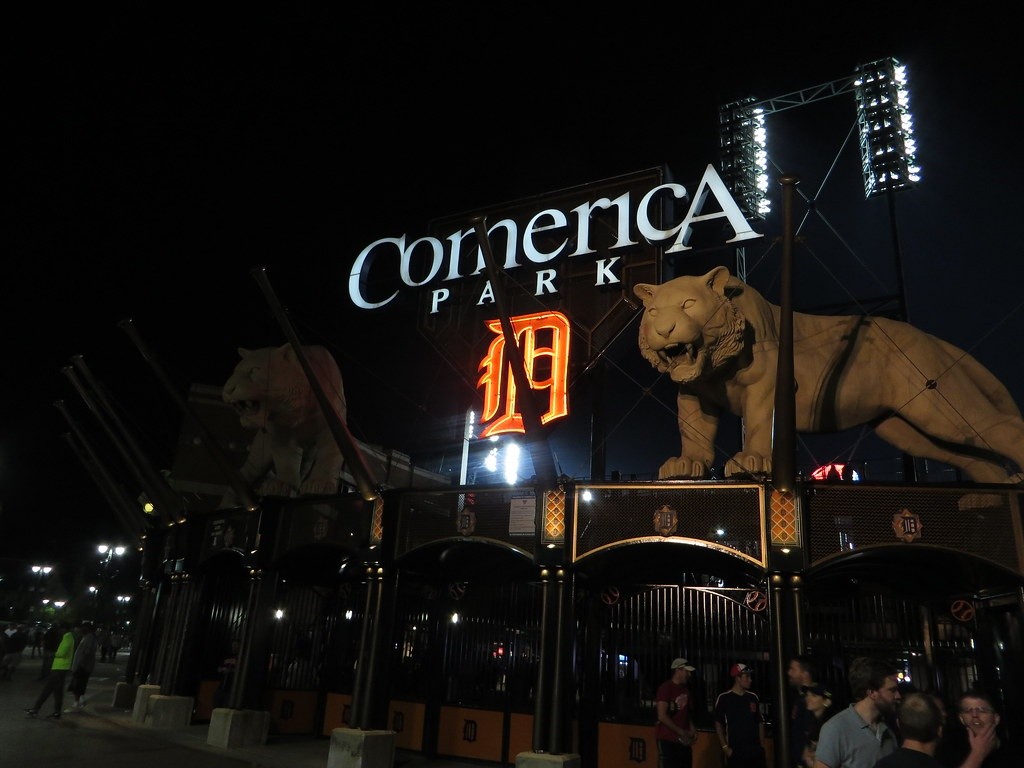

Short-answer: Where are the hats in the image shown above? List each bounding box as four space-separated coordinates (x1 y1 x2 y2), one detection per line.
731 663 754 676
801 682 833 699
671 658 696 671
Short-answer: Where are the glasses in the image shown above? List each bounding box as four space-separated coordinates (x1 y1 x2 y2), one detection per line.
961 707 993 714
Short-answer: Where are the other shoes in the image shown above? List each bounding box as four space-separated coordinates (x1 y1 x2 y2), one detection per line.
63 703 85 713
48 713 65 718
23 708 38 717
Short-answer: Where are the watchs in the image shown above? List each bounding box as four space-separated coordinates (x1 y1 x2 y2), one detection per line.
720 744 728 749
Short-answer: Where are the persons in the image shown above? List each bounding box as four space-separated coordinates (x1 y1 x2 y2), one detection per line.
787 652 845 768
711 661 769 768
796 682 844 768
63 621 98 714
0 609 132 681
654 657 699 768
813 648 1024 768
24 621 75 719
208 639 240 708
